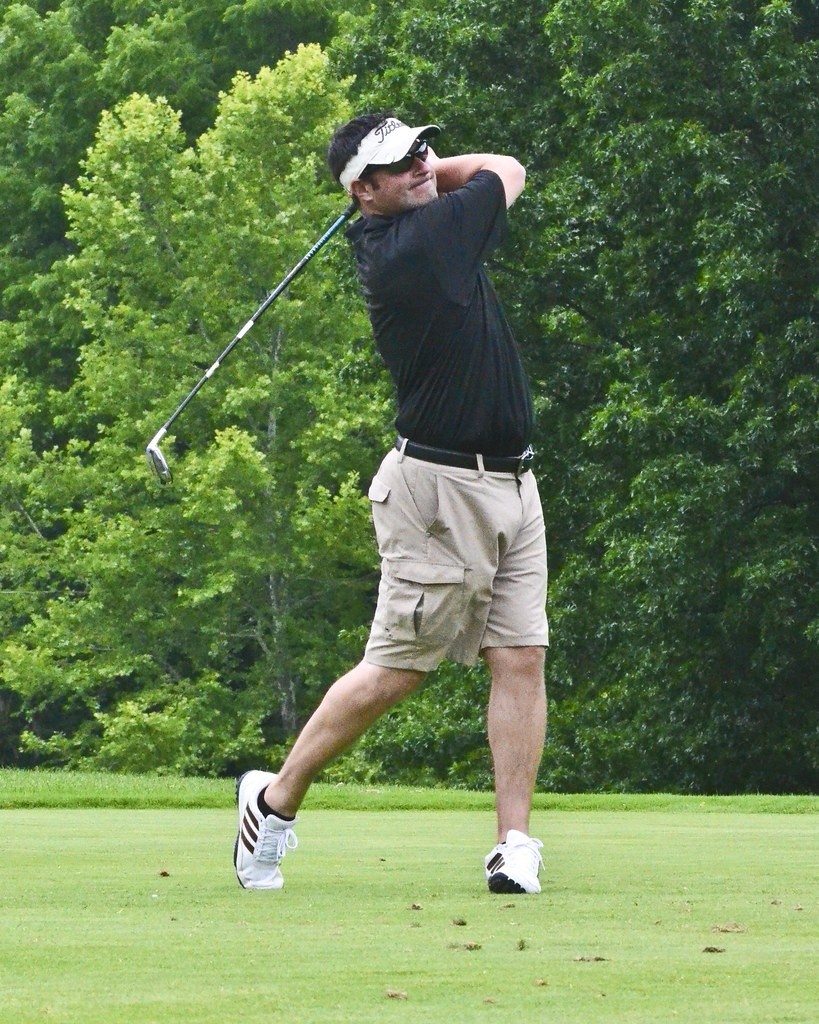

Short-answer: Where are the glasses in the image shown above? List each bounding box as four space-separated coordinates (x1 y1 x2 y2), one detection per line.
385 139 428 175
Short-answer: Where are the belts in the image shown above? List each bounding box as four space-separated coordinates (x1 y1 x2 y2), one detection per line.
396 435 535 475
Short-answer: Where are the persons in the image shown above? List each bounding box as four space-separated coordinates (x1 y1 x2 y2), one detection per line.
232 114 549 893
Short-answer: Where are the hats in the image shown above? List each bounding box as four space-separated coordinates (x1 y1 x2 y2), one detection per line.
339 118 441 198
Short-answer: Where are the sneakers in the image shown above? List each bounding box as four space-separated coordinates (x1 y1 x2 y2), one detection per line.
484 829 546 894
233 769 299 891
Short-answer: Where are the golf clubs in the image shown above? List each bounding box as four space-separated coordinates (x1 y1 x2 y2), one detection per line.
143 205 356 487
